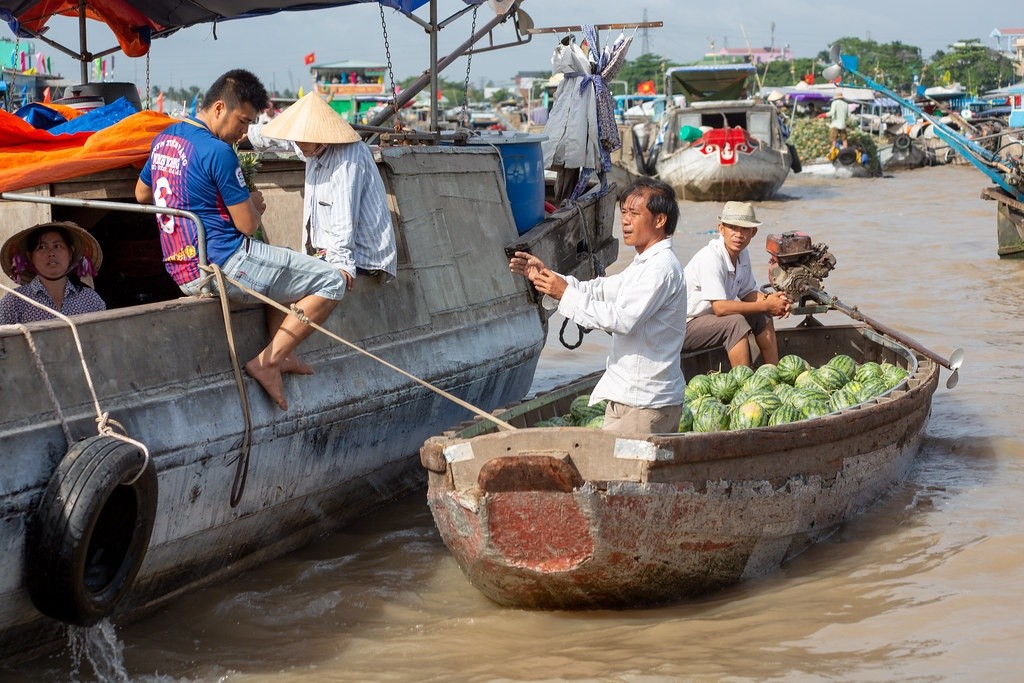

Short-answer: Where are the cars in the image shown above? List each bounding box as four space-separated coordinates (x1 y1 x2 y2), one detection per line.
443 98 526 132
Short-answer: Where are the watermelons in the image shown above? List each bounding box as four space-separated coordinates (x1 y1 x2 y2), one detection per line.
532 395 606 429
678 356 909 432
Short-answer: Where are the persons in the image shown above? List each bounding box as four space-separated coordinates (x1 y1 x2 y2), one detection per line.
135 70 346 410
318 69 378 83
684 201 789 368
0 221 107 325
261 90 398 292
508 176 686 436
816 92 848 155
804 103 822 117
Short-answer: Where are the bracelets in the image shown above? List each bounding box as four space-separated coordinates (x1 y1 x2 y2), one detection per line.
763 291 773 300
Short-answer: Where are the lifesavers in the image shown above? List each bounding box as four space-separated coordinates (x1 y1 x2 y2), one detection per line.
20 433 159 625
787 143 803 174
838 148 856 165
894 133 911 150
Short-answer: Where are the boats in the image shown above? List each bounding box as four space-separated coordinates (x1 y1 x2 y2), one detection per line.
819 43 1024 260
657 65 797 202
418 228 965 610
1 0 628 651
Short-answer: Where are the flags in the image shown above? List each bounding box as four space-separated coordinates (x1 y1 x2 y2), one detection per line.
36 52 46 74
20 51 25 72
47 56 51 73
436 88 441 100
111 55 115 80
637 78 656 95
10 49 15 70
44 87 50 102
91 57 106 81
305 53 315 65
804 72 815 85
1005 96 1020 106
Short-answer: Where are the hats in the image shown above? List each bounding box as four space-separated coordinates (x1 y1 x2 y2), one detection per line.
831 92 844 100
0 221 103 285
717 200 763 227
258 90 363 144
767 91 784 101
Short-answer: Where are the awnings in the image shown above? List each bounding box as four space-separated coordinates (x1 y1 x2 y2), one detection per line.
0 80 6 90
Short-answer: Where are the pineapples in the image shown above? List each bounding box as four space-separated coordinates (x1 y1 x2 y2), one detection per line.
786 117 879 160
233 144 262 193
